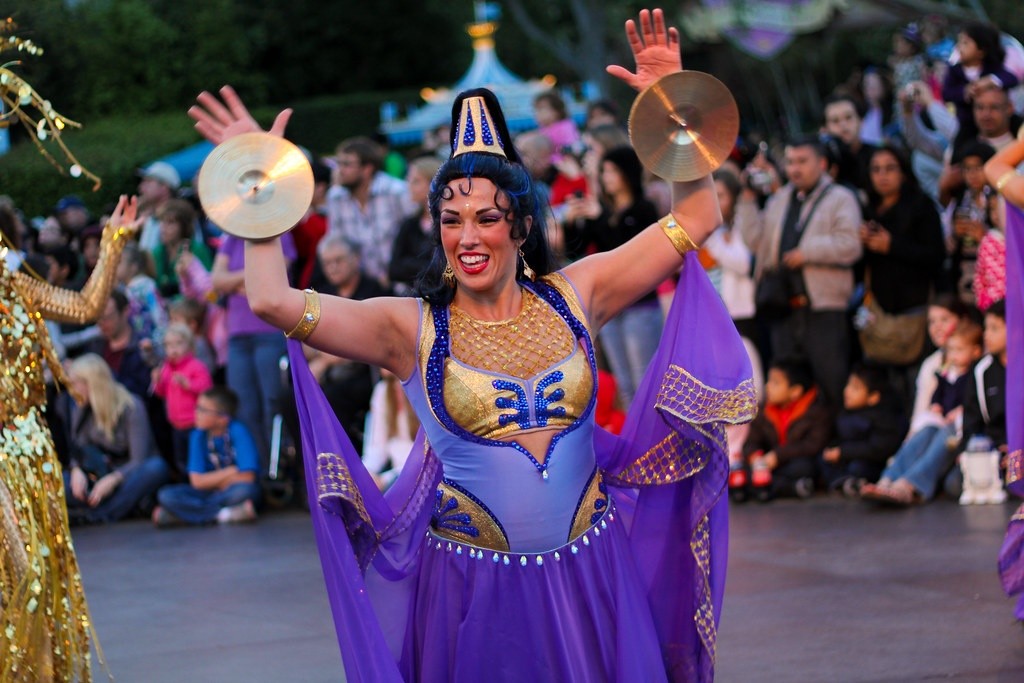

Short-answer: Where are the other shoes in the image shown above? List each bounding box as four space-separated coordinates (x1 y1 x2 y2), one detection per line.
153 507 182 528
228 498 255 524
858 482 916 508
729 465 774 502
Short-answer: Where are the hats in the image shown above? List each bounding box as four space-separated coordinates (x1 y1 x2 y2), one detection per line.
139 161 180 190
51 196 85 213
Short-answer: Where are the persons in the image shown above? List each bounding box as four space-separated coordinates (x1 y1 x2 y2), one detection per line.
188 9 756 683
0 14 1022 525
0 194 146 683
985 122 1024 624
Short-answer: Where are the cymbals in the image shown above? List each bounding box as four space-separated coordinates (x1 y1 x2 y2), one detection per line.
198 133 315 240
628 70 740 183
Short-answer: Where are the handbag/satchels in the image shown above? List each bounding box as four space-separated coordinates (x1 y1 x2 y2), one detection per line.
857 293 926 364
754 265 811 363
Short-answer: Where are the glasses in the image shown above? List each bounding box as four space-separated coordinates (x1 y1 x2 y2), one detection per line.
96 311 117 326
195 407 222 418
